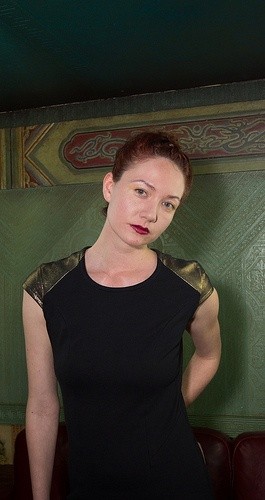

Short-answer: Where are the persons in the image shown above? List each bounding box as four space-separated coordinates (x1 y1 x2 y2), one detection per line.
20 130 221 499
